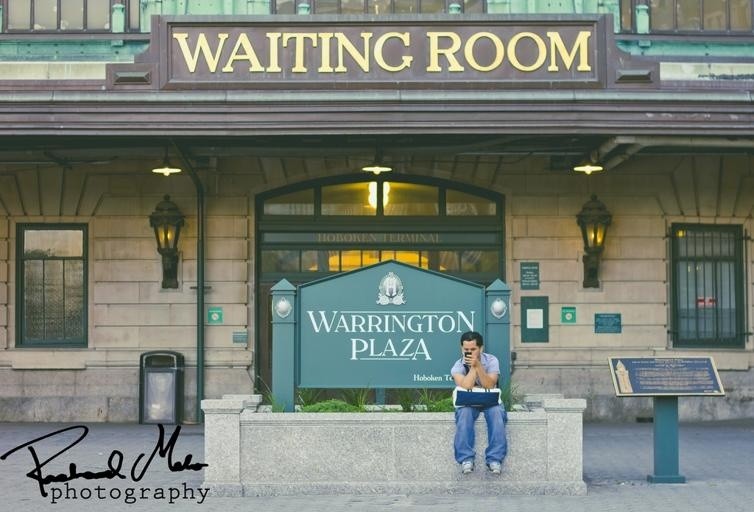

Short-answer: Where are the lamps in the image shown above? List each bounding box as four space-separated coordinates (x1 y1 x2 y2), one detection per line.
147 142 183 291
361 141 394 176
575 145 612 290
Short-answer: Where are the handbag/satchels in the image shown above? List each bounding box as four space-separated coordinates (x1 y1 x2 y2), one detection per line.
452 386 503 408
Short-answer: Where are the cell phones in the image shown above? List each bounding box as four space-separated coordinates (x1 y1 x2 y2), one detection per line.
465 352 472 357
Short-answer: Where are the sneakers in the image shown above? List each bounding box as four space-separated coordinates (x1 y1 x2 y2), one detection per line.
486 461 501 475
461 459 474 474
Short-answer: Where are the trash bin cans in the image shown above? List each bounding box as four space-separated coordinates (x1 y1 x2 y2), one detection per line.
139 350 184 424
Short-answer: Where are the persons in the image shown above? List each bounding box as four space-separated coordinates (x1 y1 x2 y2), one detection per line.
450 331 507 475
